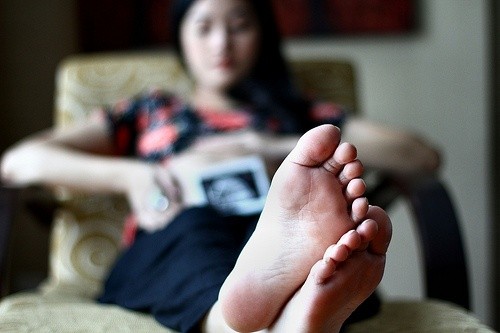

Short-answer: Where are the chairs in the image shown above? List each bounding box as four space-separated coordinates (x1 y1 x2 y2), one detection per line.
1 52 498 332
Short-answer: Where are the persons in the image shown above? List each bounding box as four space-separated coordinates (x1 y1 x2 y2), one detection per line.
0 0 442 333
